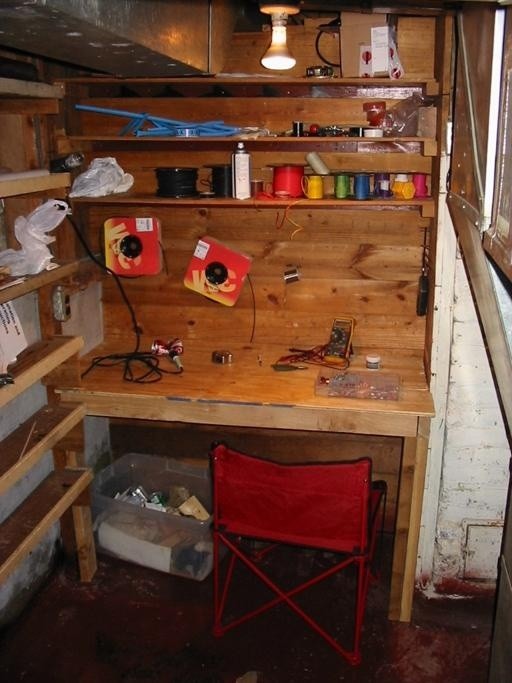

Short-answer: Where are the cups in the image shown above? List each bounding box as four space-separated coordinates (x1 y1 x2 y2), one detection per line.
306 65 334 76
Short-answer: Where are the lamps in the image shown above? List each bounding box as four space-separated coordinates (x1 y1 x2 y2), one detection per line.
261 0 300 71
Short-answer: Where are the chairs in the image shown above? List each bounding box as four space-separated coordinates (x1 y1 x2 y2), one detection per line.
209 443 387 667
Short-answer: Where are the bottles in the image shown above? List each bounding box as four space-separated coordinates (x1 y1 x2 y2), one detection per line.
293 120 303 137
230 143 251 201
366 354 381 370
50 152 84 173
251 180 263 197
302 174 428 200
52 285 70 322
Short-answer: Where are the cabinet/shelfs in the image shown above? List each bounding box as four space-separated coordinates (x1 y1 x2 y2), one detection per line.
56 76 452 218
0 77 97 584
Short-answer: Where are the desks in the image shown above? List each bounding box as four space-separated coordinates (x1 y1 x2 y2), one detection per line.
42 343 440 624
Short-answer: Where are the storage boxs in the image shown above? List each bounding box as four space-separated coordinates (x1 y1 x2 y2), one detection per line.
91 452 234 582
